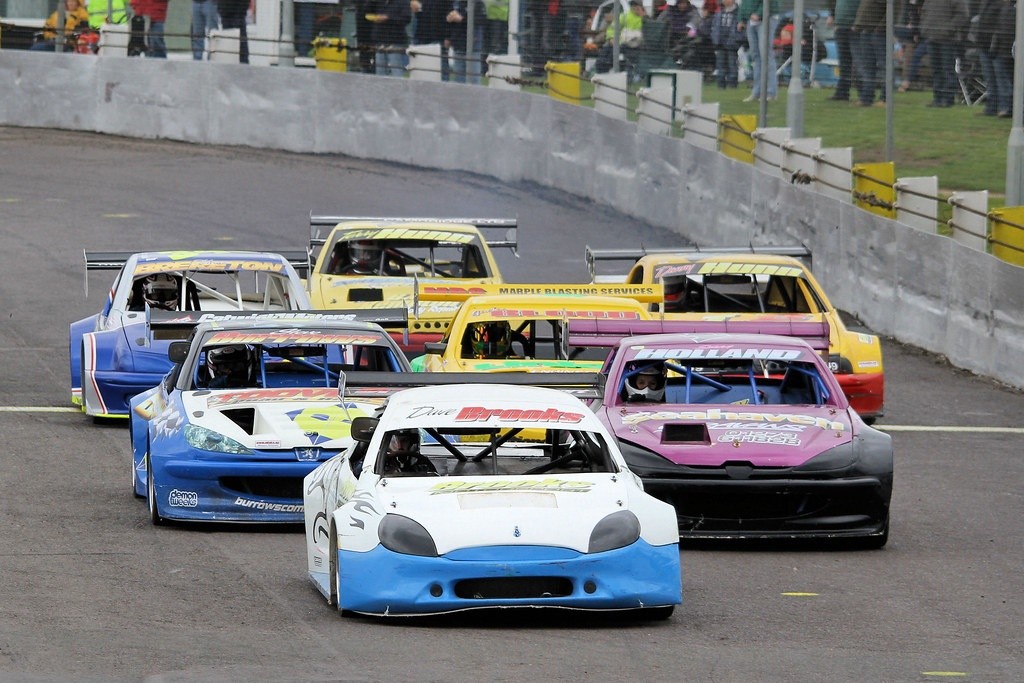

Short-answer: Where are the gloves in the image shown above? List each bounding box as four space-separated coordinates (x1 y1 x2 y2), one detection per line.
208 373 229 389
335 259 349 274
628 394 648 402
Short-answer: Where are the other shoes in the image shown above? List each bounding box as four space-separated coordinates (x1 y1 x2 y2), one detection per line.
676 58 685 66
897 85 922 92
825 96 886 107
743 95 778 102
926 101 952 108
975 110 1011 118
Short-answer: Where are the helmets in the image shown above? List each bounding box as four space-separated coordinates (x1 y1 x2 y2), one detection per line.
207 342 252 383
655 275 688 309
472 321 510 358
348 239 379 274
388 428 421 469
624 361 668 401
142 272 177 312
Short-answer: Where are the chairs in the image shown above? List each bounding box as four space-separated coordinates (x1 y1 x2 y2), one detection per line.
128 274 201 311
956 41 989 105
511 330 529 357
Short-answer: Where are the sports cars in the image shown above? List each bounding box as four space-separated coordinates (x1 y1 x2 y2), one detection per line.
302 209 520 355
570 317 898 551
584 243 886 415
65 252 314 417
127 310 414 532
407 274 658 374
302 372 682 625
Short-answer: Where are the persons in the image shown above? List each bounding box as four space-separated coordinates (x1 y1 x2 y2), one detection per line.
354 426 438 479
468 321 511 359
31 0 90 51
625 362 667 403
346 241 382 275
190 0 250 63
659 275 688 311
141 274 179 310
354 0 490 85
581 0 1017 117
129 0 168 59
206 344 252 388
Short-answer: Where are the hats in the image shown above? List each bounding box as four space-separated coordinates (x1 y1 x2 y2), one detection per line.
603 2 623 13
784 24 793 33
629 0 643 6
